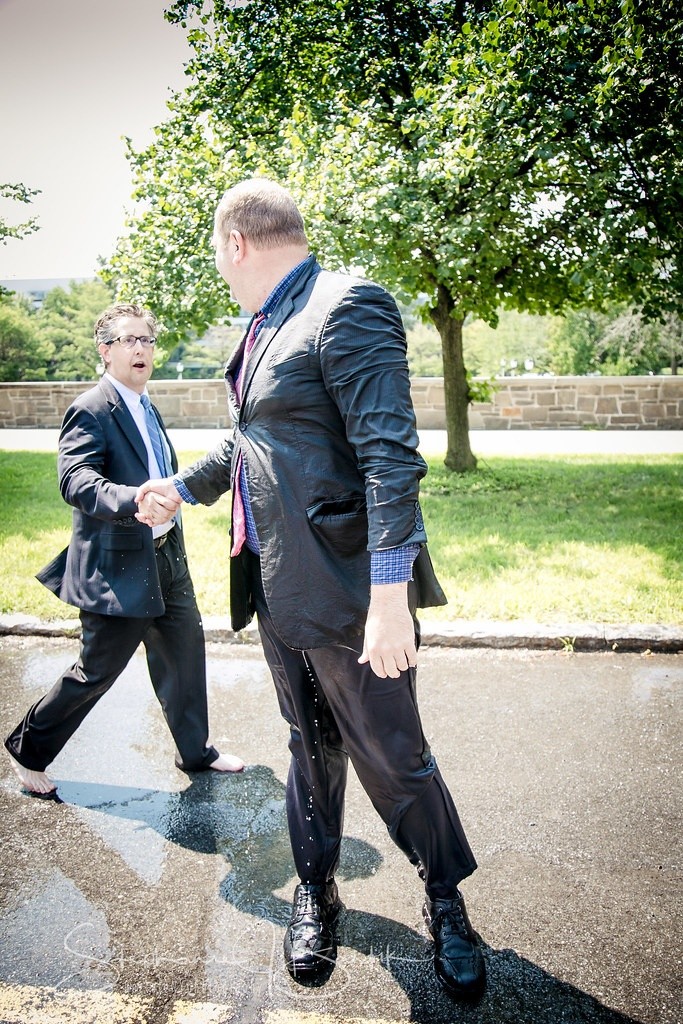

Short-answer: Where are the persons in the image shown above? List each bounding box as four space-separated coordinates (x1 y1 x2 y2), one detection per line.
135 179 488 1004
4 303 243 793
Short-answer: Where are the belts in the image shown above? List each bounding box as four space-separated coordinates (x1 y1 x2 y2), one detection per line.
153 533 168 549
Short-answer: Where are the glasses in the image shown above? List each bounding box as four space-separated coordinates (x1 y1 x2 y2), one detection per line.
105 335 157 347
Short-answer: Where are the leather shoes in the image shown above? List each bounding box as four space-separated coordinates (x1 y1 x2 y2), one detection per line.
284 879 341 971
421 887 485 1003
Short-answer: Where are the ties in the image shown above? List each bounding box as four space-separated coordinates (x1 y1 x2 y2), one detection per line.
140 394 181 530
231 311 267 559
